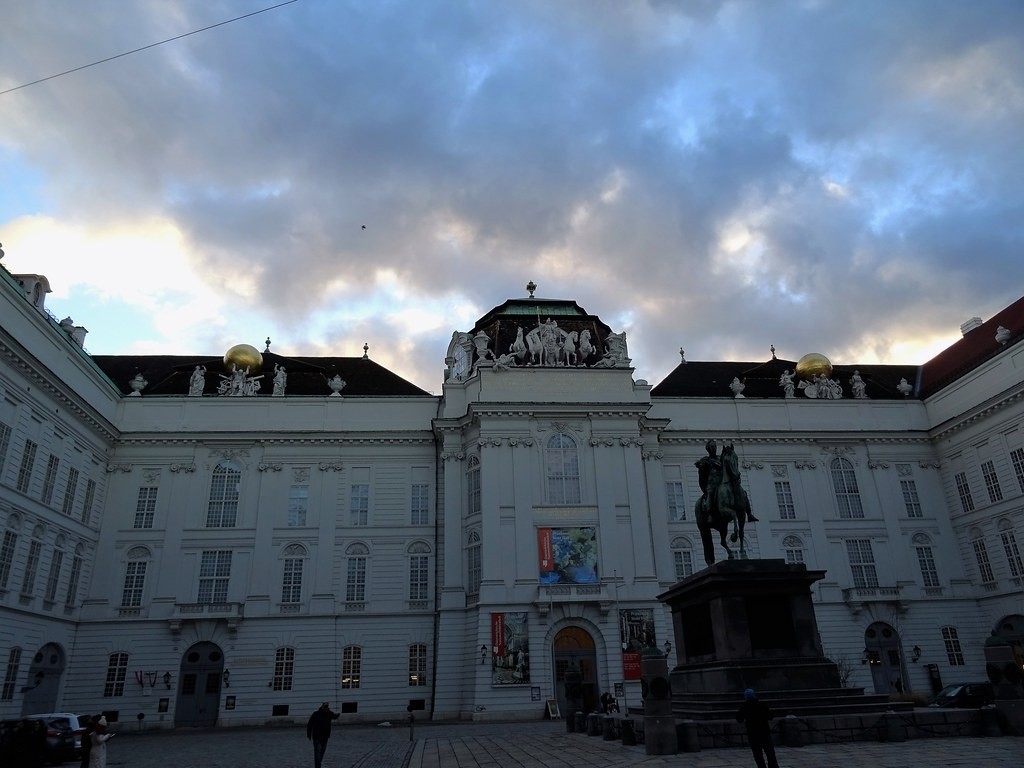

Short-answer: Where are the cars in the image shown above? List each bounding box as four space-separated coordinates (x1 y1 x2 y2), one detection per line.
926 681 996 709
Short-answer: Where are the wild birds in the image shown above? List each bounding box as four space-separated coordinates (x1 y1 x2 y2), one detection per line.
362 225 366 230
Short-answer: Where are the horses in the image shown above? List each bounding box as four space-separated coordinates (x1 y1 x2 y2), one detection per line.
509 327 596 367
694 443 749 566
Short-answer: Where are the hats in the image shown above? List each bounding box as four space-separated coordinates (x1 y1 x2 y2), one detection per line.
91 714 100 722
745 689 754 697
98 716 107 726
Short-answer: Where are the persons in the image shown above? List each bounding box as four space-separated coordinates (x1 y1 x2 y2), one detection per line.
965 685 976 696
540 318 557 343
849 370 868 397
190 365 207 393
80 714 112 768
232 363 250 395
602 692 619 714
694 440 759 522
779 369 796 389
307 702 341 768
273 363 287 388
895 678 907 695
814 372 832 399
735 689 781 768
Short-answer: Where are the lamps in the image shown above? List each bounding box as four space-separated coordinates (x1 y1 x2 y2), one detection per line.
20 670 46 693
162 672 170 689
663 640 673 658
481 645 487 665
222 669 230 688
863 647 872 664
912 644 922 663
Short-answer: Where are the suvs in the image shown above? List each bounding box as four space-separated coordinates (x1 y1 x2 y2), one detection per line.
14 712 93 761
